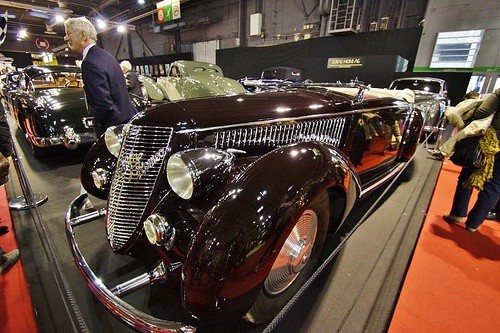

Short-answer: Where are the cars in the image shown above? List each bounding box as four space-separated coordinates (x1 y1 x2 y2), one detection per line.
1 60 303 161
389 77 450 141
64 82 424 333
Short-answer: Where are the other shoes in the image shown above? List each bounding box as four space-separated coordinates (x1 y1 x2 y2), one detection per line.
0 248 20 275
468 225 477 235
443 212 460 225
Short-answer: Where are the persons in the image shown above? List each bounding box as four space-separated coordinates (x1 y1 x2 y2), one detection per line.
349 111 404 167
0 17 138 276
442 88 500 232
119 60 144 112
